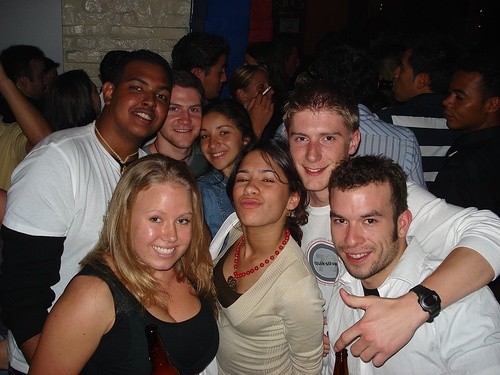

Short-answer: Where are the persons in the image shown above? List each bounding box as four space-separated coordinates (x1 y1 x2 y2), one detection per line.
28 153 220 375
0 49 173 375
172 31 230 106
232 35 428 191
326 155 500 375
194 98 254 238
142 67 213 179
0 45 129 370
376 46 464 190
428 62 500 304
205 139 323 375
208 84 500 367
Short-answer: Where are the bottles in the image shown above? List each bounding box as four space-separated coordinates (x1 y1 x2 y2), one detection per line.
333 349 349 375
144 327 181 375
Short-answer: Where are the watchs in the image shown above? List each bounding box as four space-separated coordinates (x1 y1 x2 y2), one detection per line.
408 284 442 323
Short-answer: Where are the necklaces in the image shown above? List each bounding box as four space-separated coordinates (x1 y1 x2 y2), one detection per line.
224 227 290 288
95 124 139 175
